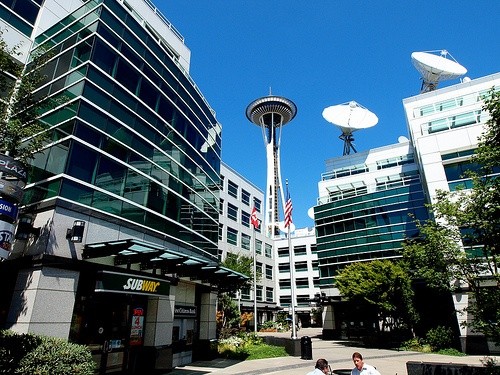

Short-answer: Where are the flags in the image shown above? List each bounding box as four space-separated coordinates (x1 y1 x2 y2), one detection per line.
284 185 293 228
251 203 259 229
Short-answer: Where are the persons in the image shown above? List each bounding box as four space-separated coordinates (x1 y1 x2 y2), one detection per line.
306 359 329 375
351 352 381 375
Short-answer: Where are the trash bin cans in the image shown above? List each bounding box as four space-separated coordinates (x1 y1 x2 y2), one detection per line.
299 336 312 360
201 338 220 361
